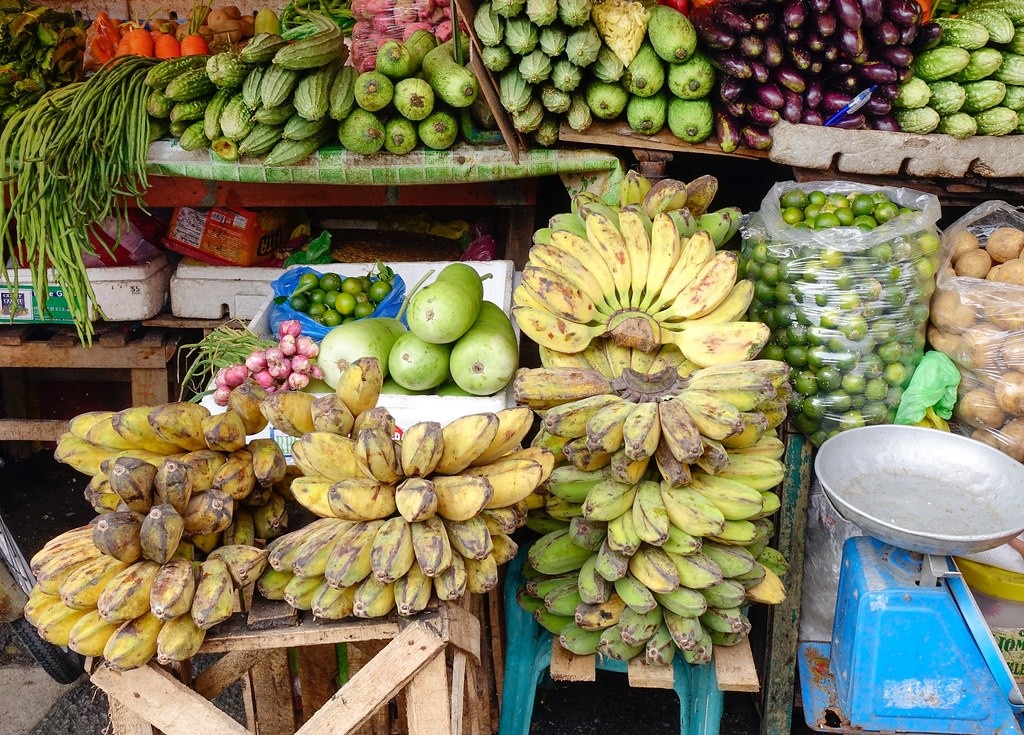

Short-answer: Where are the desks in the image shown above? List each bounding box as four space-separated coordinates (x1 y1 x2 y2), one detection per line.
0 306 203 439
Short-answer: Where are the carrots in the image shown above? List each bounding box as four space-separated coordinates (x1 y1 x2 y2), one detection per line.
92 13 207 67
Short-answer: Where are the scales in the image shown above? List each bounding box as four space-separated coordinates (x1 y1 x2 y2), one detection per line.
794 422 1024 735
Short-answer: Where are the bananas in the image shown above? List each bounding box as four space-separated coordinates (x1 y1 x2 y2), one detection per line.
23 383 287 670
259 358 554 618
511 169 793 667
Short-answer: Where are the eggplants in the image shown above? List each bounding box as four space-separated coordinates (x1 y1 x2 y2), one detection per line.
689 0 943 155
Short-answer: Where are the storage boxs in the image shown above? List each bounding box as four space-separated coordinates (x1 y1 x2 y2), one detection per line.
0 247 169 325
204 260 525 465
171 255 285 320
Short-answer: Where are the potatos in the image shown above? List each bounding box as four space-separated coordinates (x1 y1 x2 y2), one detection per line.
149 6 255 42
929 226 1024 464
349 0 466 75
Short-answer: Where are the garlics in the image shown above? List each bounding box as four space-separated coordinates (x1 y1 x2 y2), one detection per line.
214 320 323 406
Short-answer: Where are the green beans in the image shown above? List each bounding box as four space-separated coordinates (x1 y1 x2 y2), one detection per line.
0 54 168 348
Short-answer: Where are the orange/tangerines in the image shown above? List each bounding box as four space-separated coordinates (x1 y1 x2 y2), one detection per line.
738 191 940 443
291 272 390 325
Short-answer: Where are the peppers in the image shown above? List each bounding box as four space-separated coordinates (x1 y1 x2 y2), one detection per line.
277 0 353 37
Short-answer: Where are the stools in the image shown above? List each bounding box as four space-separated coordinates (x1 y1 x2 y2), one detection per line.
497 528 748 735
